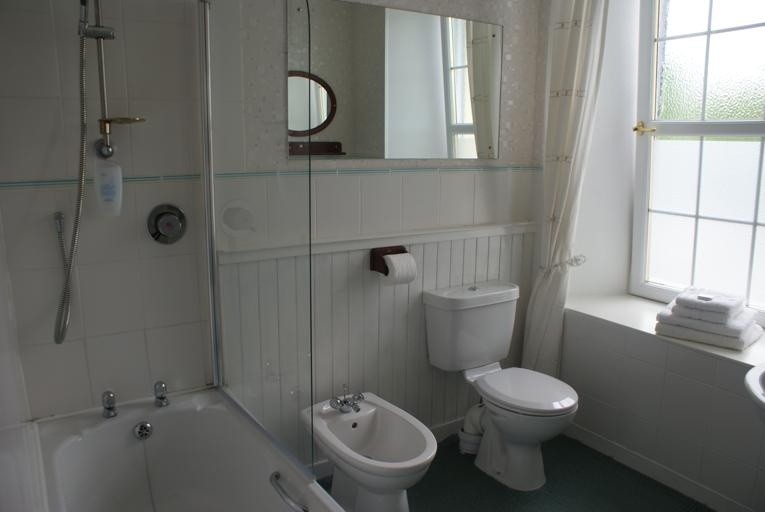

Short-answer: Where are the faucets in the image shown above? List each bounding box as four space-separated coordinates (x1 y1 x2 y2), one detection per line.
330 391 366 415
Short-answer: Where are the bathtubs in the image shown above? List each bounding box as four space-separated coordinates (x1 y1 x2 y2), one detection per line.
34 385 346 512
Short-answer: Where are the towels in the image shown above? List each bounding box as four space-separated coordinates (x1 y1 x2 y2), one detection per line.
654 284 765 351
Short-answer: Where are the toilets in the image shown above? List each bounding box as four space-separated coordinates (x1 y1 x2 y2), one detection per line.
422 279 580 493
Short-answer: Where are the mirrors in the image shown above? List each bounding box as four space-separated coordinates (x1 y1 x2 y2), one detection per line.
287 69 338 135
284 1 503 163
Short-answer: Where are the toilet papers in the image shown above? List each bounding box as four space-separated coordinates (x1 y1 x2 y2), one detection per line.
382 252 417 285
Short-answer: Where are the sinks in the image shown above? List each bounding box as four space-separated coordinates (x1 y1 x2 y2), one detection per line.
301 391 438 512
